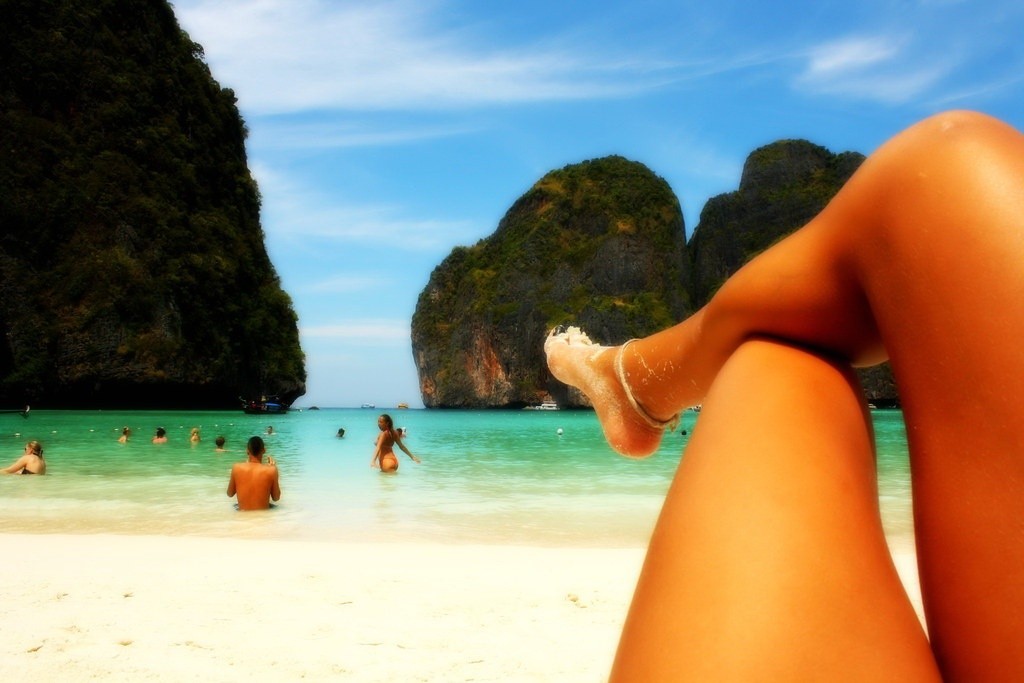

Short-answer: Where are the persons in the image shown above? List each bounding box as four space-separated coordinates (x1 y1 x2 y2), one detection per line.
543 111 1024 683
0 440 46 475
371 414 420 472
191 428 200 441
118 427 129 442
152 427 167 444
396 428 406 438
267 426 272 434
227 436 281 512
336 427 345 437
215 437 226 452
